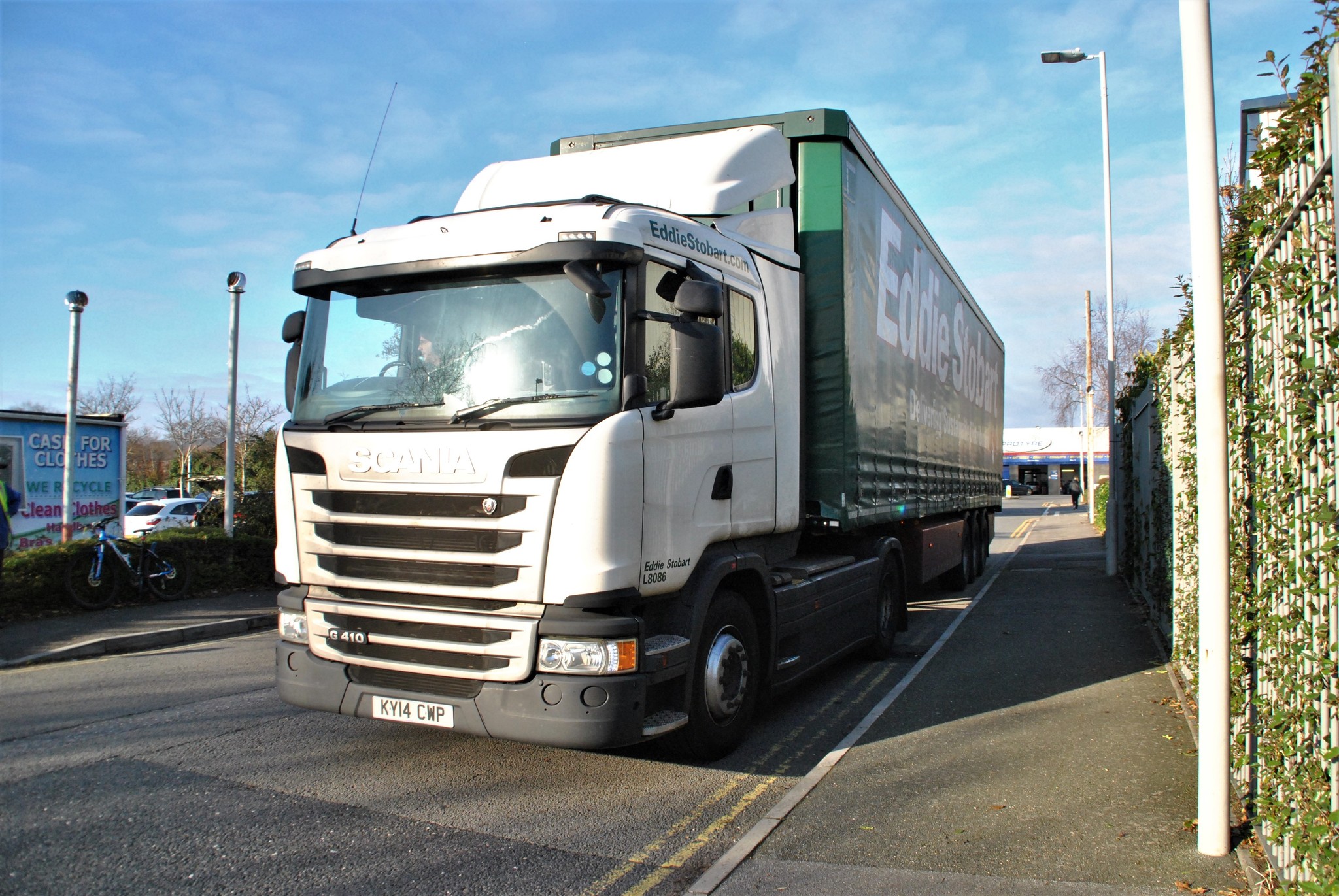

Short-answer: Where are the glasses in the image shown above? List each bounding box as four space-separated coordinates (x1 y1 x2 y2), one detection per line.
420 340 429 345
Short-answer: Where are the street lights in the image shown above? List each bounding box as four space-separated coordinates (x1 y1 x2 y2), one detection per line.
1039 47 1121 579
58 290 90 545
223 272 247 582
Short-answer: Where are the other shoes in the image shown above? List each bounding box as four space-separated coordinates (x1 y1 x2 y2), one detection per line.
1073 506 1075 508
1075 509 1078 510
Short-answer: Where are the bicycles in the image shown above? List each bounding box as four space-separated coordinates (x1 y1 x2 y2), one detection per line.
64 515 190 610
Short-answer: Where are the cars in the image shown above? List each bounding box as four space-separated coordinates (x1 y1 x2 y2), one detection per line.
125 475 275 539
1001 479 1038 495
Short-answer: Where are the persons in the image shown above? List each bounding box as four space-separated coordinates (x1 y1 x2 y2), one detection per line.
1060 480 1065 487
1069 476 1083 510
0 456 21 629
411 328 455 404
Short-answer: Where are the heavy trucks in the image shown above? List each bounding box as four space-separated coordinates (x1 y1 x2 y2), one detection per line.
271 106 1009 757
